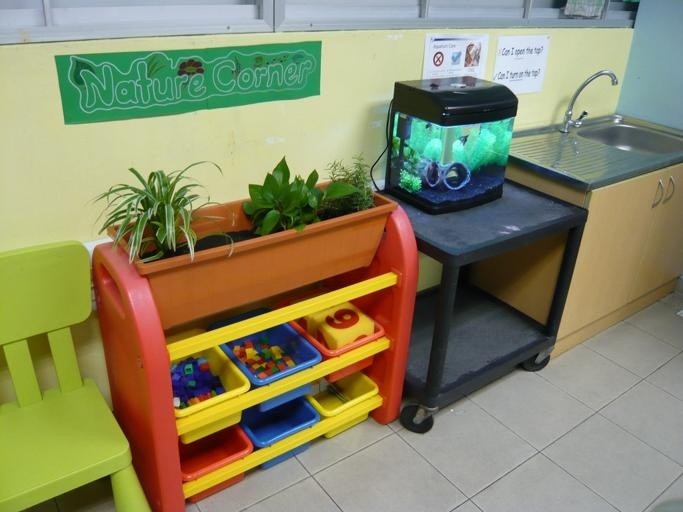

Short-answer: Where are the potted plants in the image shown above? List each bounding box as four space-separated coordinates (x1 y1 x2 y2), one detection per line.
83 151 398 332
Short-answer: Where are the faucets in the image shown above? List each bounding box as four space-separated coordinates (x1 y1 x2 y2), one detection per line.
559 69 618 133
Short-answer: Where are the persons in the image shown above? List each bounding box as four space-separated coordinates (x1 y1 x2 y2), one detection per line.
475 49 480 62
464 43 475 68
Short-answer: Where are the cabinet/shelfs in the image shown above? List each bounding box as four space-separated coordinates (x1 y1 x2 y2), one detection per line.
472 161 682 361
379 177 590 434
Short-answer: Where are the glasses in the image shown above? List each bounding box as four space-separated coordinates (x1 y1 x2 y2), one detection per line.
424 160 471 191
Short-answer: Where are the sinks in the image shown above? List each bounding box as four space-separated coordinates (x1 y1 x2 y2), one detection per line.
576 115 683 154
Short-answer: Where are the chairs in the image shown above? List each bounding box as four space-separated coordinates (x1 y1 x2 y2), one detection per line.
1 243 155 512
